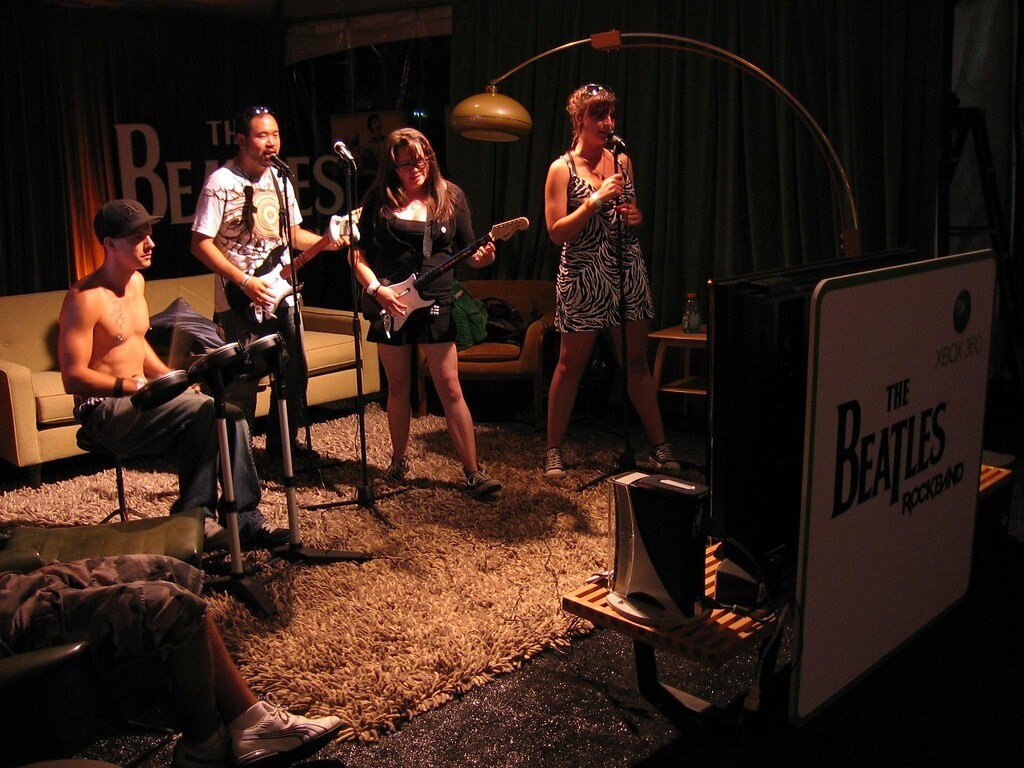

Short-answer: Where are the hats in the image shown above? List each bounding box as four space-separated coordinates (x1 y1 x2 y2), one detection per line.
92 198 165 245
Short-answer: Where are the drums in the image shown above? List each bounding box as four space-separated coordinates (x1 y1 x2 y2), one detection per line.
130 370 190 411
188 334 289 383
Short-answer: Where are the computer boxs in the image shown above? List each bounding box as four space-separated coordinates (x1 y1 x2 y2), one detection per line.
609 471 713 625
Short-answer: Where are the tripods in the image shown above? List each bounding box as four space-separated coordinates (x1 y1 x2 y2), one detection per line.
575 138 678 492
276 150 413 530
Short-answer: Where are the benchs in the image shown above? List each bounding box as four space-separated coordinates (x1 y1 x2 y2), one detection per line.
561 461 1013 732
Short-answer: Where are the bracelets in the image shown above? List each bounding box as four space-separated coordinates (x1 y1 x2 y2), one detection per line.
240 275 251 290
591 193 602 207
137 378 147 390
114 377 123 397
367 280 381 297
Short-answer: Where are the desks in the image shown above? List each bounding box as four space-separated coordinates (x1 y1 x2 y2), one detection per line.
648 316 710 397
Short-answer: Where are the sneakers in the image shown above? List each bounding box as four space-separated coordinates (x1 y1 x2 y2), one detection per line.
203 516 226 552
462 464 501 495
647 443 678 469
229 692 344 768
173 726 236 768
543 447 565 477
248 521 291 546
384 456 410 482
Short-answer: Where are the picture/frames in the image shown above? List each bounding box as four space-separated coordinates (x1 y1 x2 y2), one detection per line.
330 110 408 177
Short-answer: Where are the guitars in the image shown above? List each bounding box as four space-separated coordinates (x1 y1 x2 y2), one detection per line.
234 207 363 325
360 216 530 341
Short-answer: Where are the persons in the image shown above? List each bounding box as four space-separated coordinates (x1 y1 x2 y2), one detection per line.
189 109 350 464
0 553 343 768
546 99 692 490
59 201 291 545
346 127 503 497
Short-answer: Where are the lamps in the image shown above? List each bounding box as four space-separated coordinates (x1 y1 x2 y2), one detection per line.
449 30 864 257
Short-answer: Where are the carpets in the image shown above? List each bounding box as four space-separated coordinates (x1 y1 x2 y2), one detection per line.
0 399 709 744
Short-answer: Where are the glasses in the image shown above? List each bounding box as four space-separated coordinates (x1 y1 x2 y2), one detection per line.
245 105 271 116
394 158 426 170
580 84 613 105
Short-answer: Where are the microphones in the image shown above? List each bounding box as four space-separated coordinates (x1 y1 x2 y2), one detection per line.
270 153 293 174
607 130 629 150
334 141 355 164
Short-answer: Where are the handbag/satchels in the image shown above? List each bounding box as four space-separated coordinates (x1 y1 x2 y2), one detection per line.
481 294 529 346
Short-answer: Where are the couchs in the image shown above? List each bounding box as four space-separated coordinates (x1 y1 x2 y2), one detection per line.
1 272 380 489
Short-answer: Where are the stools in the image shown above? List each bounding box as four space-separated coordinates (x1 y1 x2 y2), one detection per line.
76 427 151 526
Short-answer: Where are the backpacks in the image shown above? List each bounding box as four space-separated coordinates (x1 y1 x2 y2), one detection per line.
449 278 488 349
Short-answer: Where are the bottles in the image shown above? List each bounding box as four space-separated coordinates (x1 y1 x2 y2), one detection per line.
682 294 702 334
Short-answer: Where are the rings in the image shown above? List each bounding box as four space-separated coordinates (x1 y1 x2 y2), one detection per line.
629 209 633 215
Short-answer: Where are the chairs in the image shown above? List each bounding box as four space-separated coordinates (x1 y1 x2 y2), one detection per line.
0 505 205 768
418 280 560 419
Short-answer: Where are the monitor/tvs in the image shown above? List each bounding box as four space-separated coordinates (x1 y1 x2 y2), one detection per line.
705 246 920 559
